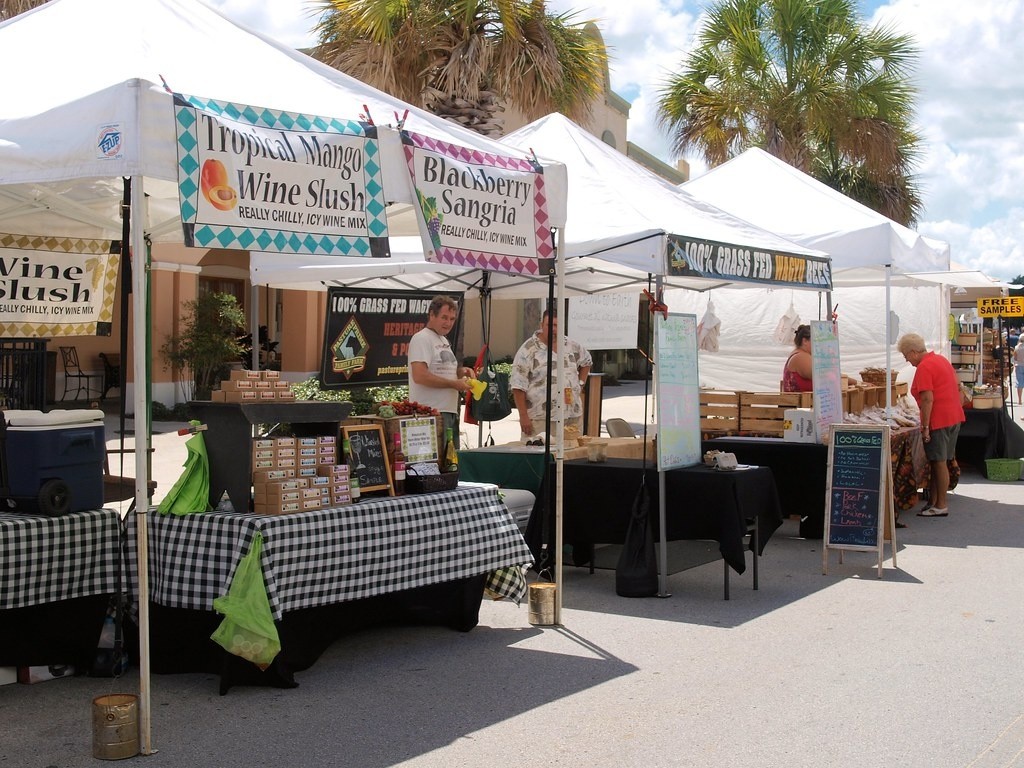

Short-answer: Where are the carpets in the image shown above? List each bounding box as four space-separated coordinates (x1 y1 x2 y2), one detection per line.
569 539 749 575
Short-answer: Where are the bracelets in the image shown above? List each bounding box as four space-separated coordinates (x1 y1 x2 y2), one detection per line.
920 425 930 430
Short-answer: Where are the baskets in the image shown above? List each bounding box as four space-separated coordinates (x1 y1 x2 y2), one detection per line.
859 370 899 385
406 463 460 492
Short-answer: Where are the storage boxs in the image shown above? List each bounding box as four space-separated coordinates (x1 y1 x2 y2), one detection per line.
955 369 977 382
985 458 1024 482
342 413 445 496
783 408 817 444
953 335 980 346
212 368 295 401
251 435 359 514
2 408 105 517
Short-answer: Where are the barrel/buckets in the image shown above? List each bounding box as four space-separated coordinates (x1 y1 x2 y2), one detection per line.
528 569 556 625
91 675 140 759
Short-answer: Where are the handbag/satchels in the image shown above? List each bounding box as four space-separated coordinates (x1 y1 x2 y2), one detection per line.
208 531 282 671
463 343 512 426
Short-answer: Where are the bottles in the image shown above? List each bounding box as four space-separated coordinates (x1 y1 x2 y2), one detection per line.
442 428 458 472
389 433 406 496
343 440 361 504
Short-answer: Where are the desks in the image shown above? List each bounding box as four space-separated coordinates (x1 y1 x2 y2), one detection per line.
455 401 1024 601
0 480 537 697
186 400 353 513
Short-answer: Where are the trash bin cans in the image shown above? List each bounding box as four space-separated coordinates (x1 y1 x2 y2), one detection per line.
13 350 58 405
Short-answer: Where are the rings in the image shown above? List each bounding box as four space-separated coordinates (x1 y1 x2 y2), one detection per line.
925 439 928 441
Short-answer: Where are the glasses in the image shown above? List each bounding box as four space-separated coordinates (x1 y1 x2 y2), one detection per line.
525 439 544 446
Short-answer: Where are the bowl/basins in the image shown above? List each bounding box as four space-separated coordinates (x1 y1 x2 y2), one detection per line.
373 404 413 417
586 444 607 463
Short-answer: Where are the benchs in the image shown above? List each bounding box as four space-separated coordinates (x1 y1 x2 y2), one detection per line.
99 352 122 401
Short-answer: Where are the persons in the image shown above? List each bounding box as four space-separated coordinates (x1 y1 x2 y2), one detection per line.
510 308 593 441
408 295 476 454
783 324 813 392
1005 327 1024 405
898 332 967 517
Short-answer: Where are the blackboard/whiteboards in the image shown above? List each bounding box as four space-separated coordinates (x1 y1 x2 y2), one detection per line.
654 309 702 472
341 424 393 493
810 318 848 445
824 423 896 552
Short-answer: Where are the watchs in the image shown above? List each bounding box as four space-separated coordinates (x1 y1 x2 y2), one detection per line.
579 380 585 385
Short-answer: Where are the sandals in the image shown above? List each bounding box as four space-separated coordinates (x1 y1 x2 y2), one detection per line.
916 506 948 517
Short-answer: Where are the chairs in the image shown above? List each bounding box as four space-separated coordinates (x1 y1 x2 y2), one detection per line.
605 418 636 438
59 345 105 404
102 448 157 502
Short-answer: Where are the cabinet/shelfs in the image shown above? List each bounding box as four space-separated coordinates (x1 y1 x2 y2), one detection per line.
950 341 978 392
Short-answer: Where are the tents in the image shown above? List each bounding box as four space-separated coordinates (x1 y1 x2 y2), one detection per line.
675 145 952 487
951 260 1024 388
494 111 833 598
0 0 569 756
